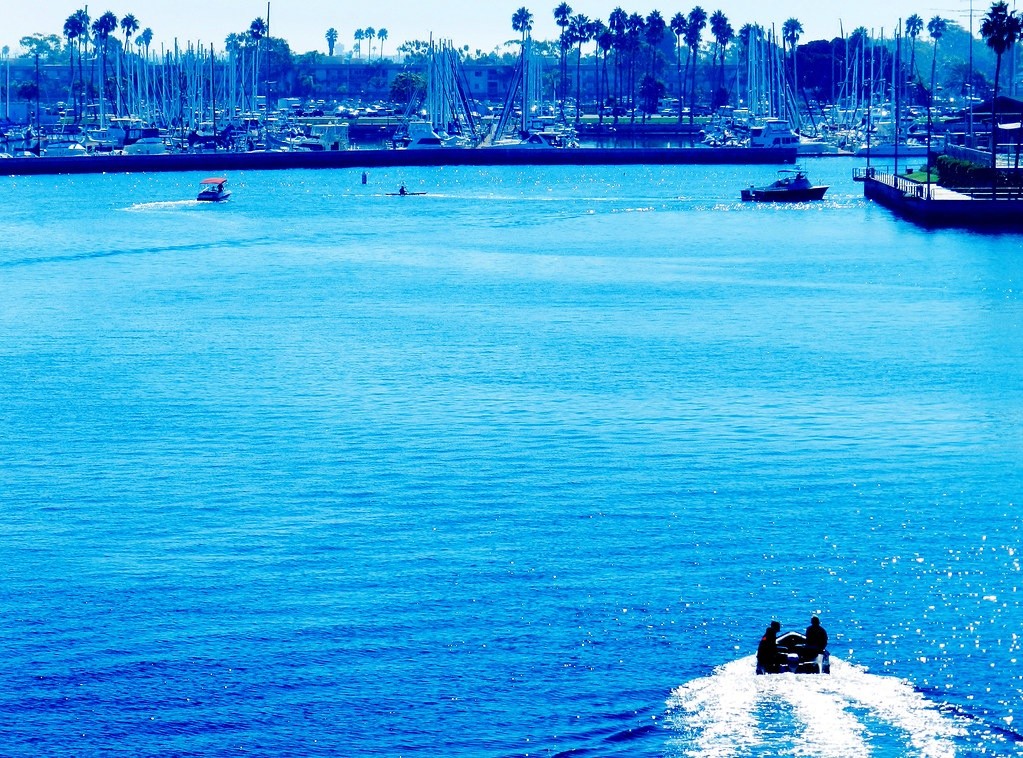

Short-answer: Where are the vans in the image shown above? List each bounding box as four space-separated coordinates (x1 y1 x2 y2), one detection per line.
493 107 504 118
512 107 521 117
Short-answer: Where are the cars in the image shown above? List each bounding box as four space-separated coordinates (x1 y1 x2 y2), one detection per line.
302 108 325 118
682 108 690 115
627 109 645 117
661 109 678 117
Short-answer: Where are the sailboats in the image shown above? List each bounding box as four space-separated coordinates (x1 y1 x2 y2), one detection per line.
0 31 581 152
700 19 944 150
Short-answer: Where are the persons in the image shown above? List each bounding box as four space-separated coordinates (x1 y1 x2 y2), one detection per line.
797 617 827 662
757 621 786 672
217 183 223 193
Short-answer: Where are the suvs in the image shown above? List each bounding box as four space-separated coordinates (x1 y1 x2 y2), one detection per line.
598 107 614 117
612 107 627 117
340 109 358 119
570 109 583 117
358 108 374 116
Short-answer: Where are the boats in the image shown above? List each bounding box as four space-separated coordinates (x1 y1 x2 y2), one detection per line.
739 165 830 205
195 179 232 203
386 191 426 195
754 631 830 677
